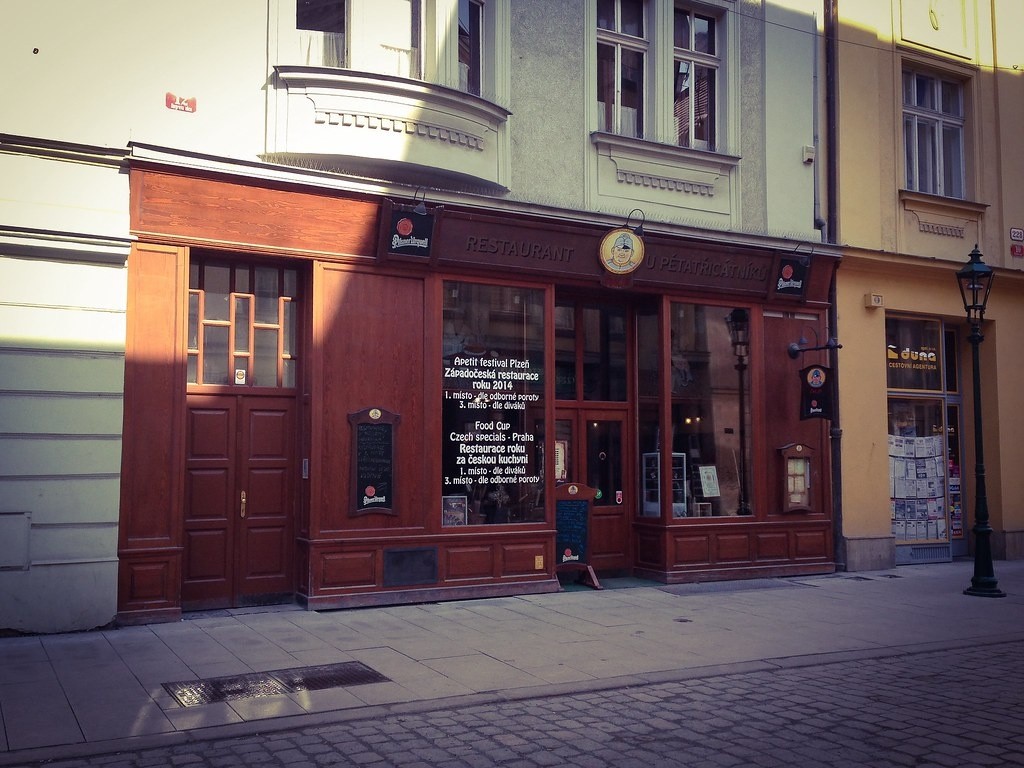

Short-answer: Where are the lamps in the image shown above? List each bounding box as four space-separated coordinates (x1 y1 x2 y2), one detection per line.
624 209 645 237
413 185 427 215
793 241 815 267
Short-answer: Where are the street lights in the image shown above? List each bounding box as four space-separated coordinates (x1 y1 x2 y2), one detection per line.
955 243 1007 597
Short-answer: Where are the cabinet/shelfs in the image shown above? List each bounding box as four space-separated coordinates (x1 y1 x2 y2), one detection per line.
642 452 686 517
